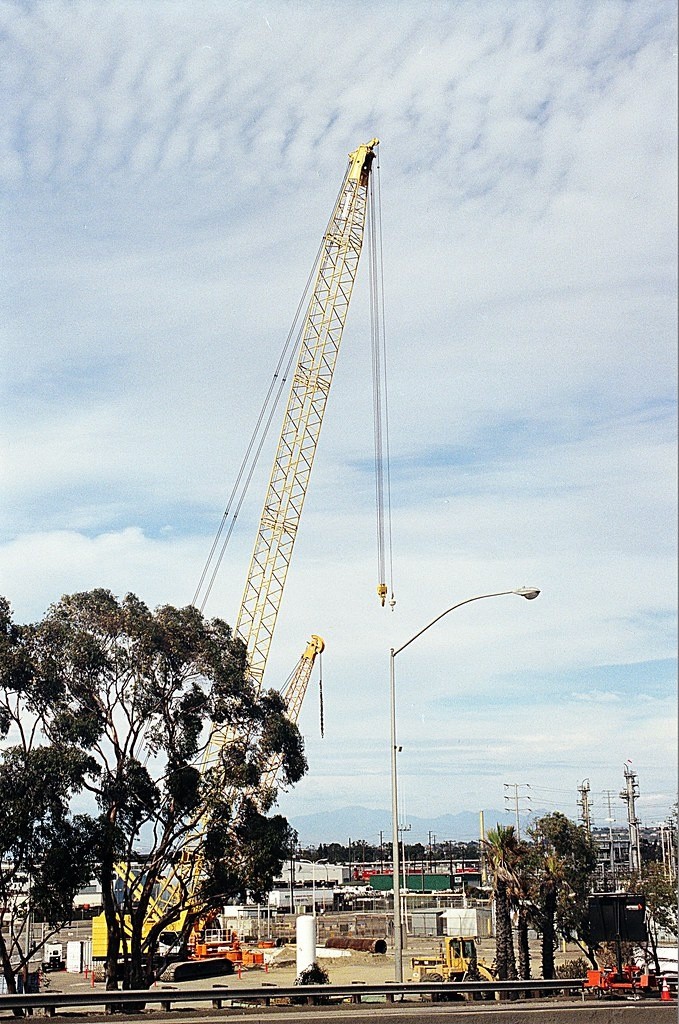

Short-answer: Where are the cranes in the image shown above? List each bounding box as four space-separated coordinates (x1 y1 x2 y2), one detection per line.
91 138 411 983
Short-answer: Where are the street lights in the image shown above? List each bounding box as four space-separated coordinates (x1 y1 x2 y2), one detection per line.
390 585 541 1000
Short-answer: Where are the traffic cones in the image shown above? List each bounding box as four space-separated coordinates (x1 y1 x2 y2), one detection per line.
658 977 674 1002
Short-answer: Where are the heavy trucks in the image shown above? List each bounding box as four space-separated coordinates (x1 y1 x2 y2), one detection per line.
267 890 345 914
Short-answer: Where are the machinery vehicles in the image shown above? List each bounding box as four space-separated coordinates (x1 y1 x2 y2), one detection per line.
412 936 502 1000
